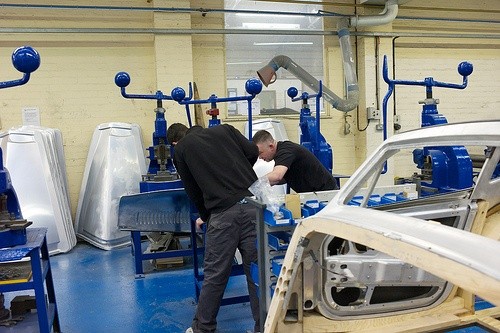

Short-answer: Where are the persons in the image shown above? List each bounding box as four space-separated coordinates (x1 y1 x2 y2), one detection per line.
168 123 268 333
252 130 339 193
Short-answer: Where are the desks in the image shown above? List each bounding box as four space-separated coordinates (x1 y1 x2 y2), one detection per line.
0 227 61 333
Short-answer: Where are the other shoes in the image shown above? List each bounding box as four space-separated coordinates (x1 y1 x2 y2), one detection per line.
185 327 193 333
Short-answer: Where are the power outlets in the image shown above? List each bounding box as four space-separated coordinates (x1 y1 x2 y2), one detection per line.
367 108 380 120
